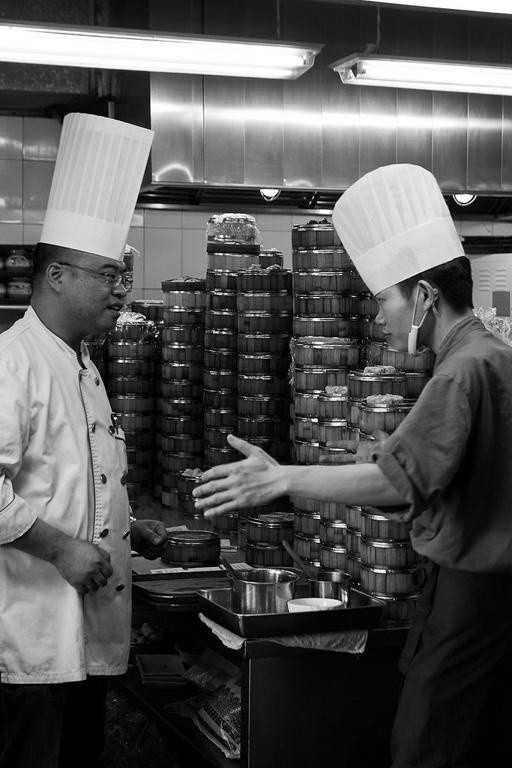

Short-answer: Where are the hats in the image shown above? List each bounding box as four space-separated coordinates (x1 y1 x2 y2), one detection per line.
332 163 466 295
37 111 154 261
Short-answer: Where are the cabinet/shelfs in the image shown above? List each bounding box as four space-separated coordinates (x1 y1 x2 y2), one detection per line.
117 595 411 768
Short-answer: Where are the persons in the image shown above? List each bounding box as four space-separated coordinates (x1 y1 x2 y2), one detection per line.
0 233 168 767
188 253 509 767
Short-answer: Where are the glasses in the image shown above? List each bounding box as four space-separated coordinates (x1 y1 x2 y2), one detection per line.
56 260 134 292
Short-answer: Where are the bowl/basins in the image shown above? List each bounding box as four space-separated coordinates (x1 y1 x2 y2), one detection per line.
285 595 343 613
224 568 300 615
306 569 353 609
